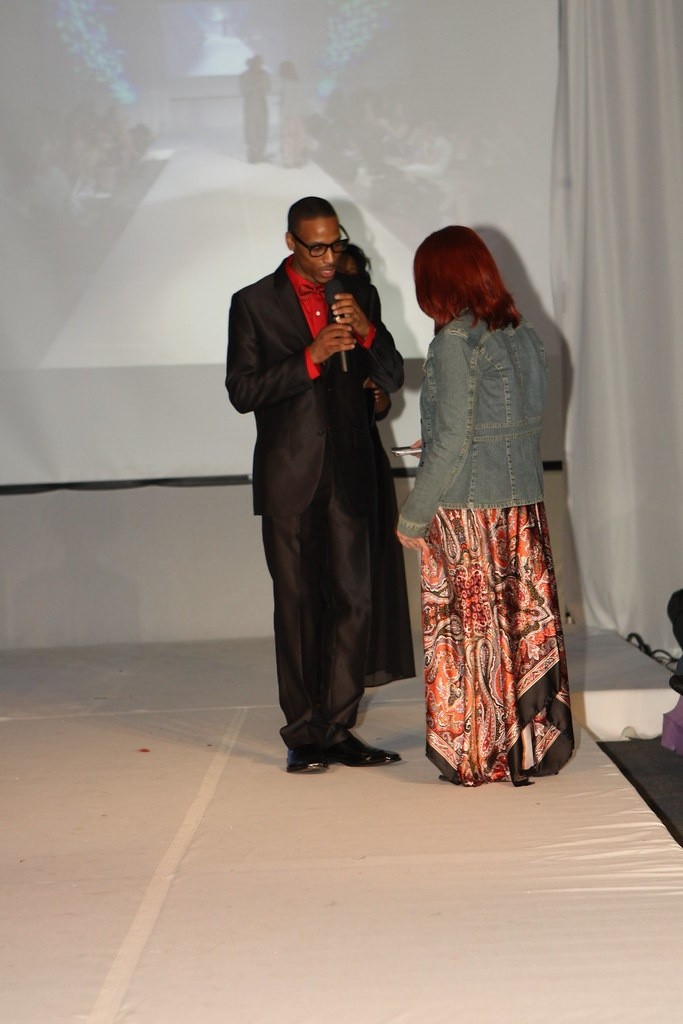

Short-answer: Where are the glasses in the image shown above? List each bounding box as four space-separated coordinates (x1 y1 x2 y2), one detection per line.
291 225 350 257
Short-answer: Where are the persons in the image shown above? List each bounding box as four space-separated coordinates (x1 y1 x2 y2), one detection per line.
398 225 575 788
307 78 453 222
226 195 416 772
236 54 304 168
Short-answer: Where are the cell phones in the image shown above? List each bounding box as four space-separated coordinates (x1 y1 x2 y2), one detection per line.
390 447 422 455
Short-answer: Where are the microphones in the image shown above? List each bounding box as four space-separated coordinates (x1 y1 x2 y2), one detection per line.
324 279 351 373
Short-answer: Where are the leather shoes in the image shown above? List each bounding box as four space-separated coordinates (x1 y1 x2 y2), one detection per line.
329 736 402 767
286 748 328 773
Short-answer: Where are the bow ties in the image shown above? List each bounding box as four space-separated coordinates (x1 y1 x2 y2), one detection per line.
299 284 326 298
